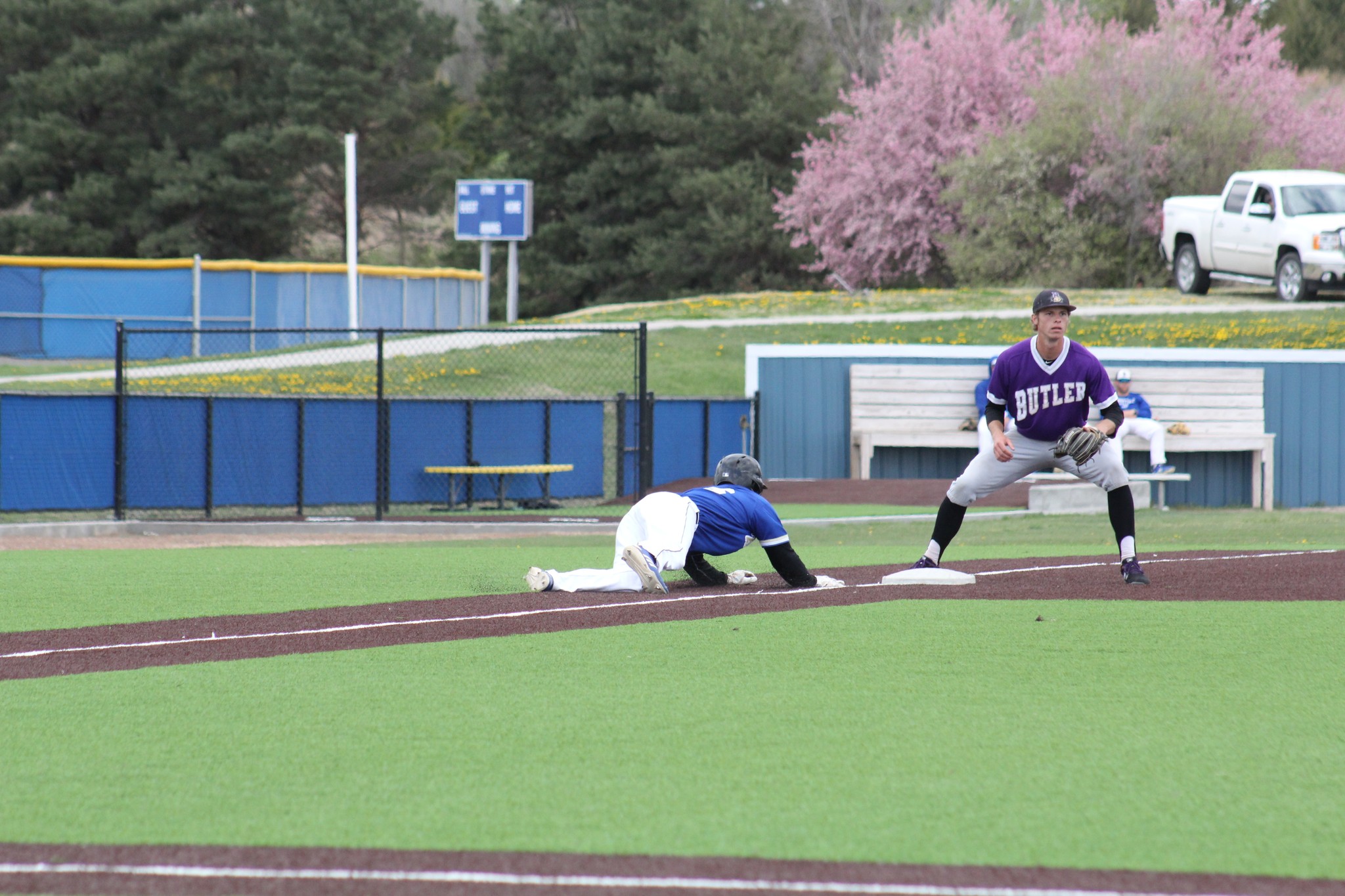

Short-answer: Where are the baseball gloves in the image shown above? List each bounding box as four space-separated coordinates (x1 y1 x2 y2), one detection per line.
1048 425 1111 474
1168 422 1190 435
958 418 977 431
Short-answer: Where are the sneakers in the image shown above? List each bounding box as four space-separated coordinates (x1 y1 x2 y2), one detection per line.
908 555 940 570
1151 463 1176 473
523 566 554 592
621 542 669 594
1121 557 1150 584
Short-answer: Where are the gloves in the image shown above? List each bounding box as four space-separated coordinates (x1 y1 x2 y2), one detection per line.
815 575 845 587
727 570 757 585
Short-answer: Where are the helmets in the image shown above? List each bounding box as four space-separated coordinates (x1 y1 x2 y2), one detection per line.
713 454 768 496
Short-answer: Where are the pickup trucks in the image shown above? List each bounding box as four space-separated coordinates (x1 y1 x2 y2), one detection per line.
1158 170 1345 301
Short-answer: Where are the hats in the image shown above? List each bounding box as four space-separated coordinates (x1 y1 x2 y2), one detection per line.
1115 369 1132 383
1032 290 1077 313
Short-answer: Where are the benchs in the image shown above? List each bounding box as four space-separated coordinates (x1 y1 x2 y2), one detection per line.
850 363 1275 511
424 464 573 511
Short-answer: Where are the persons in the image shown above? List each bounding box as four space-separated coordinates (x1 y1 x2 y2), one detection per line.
910 288 1151 586
1101 368 1178 475
521 453 848 593
974 355 1021 456
